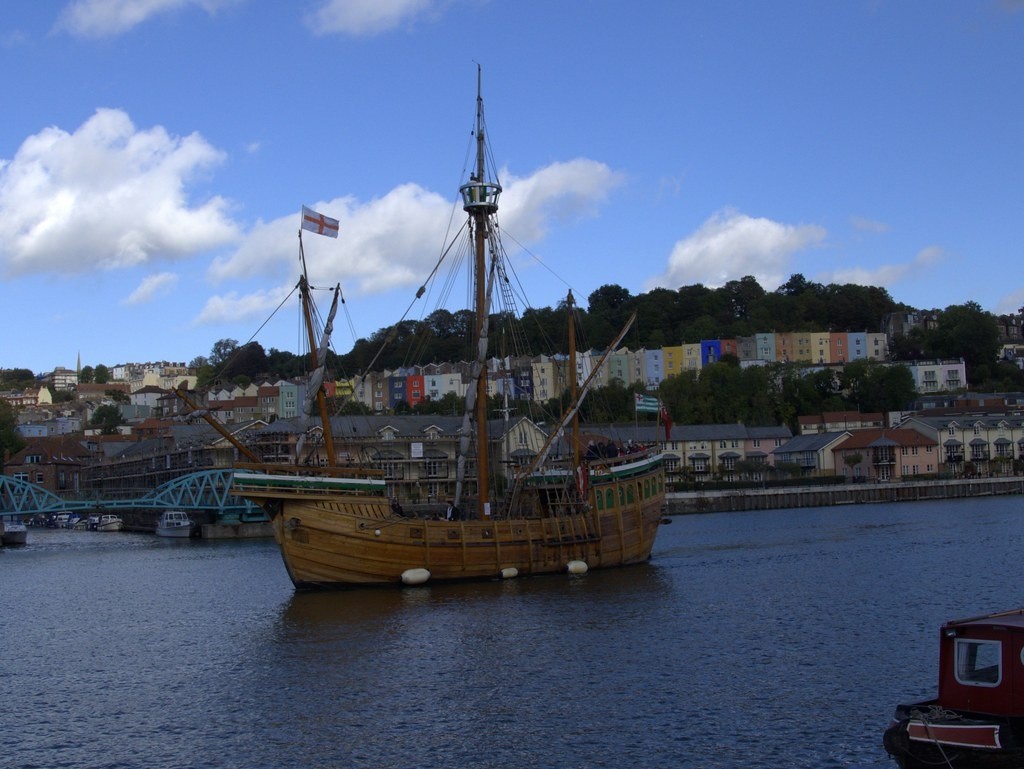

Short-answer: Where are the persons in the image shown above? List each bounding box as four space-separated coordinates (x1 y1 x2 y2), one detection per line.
446 500 459 522
432 511 449 521
587 436 648 470
391 499 409 521
954 468 982 479
886 472 890 483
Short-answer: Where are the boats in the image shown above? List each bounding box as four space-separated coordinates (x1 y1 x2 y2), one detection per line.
157 512 192 538
4 511 124 544
169 52 672 590
880 607 1024 769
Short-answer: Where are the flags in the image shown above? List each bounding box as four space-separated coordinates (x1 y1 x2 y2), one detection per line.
661 404 673 442
636 392 660 412
577 462 587 500
302 205 339 239
